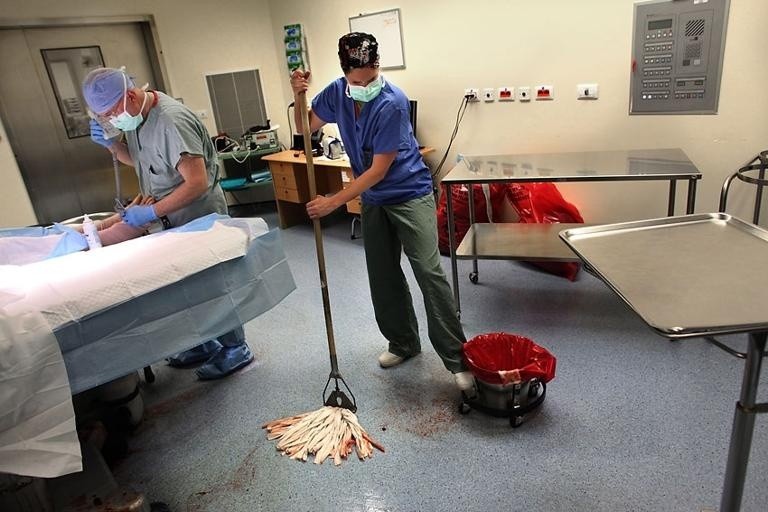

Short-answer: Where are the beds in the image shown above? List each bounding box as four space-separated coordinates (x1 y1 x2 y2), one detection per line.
0 209 274 427
1 209 128 264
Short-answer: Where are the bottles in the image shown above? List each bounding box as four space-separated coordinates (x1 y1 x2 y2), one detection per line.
82 214 101 250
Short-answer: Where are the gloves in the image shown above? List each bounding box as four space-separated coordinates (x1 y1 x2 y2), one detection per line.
121 205 158 228
89 118 114 149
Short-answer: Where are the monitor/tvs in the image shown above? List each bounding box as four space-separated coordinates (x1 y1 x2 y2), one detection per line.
407 100 417 138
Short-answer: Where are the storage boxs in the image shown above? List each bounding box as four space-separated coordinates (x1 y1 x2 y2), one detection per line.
252 130 278 149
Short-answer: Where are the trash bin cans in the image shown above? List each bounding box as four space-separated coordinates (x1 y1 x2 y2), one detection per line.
458 333 557 428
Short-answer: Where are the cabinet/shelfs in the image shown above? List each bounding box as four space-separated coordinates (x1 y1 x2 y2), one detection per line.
442 148 703 326
215 147 283 205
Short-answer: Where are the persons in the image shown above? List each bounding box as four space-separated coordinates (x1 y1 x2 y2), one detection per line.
0 192 156 266
289 32 476 391
80 68 254 382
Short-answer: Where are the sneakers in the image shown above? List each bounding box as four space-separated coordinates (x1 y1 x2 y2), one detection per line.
451 368 475 392
169 339 224 369
194 340 254 381
378 344 421 368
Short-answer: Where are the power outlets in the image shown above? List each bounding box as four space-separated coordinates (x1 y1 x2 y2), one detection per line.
464 89 480 103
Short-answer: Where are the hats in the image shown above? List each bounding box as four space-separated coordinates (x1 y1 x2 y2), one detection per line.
337 31 380 73
82 67 134 114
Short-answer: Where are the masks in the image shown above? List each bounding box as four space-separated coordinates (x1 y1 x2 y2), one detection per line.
348 79 381 103
109 101 146 132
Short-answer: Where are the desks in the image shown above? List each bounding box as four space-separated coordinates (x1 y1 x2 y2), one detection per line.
262 147 435 237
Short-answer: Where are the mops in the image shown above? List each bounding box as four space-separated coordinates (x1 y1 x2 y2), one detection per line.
258 93 387 469
